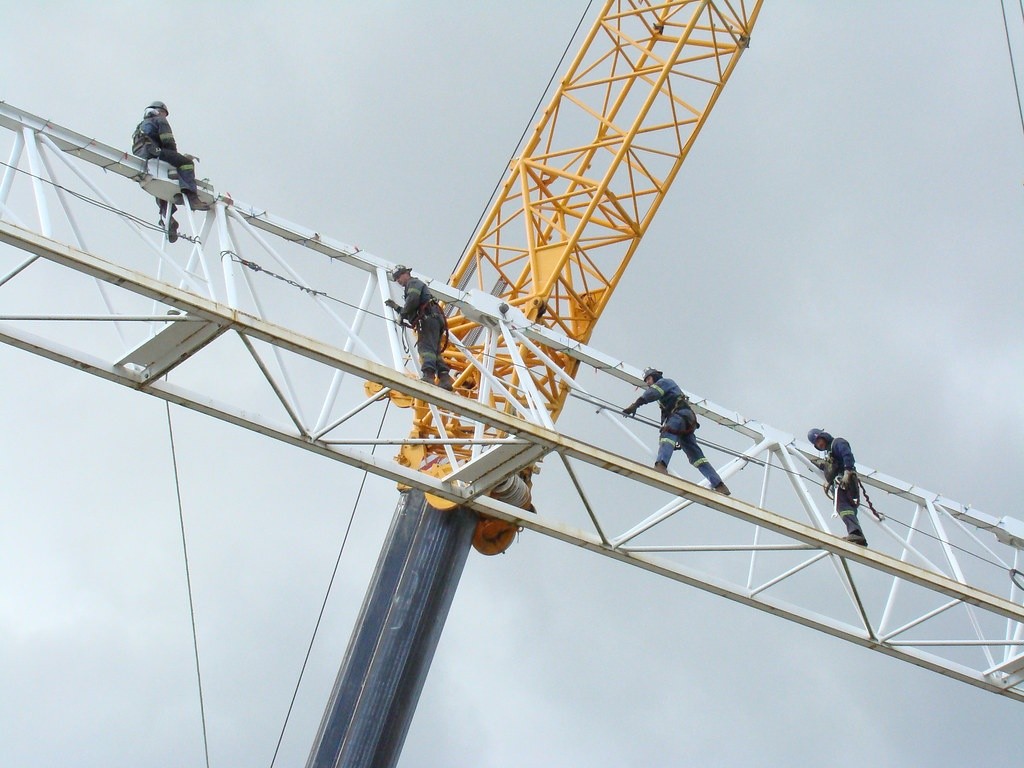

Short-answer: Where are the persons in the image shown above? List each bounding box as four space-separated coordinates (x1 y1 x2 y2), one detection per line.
622 367 731 496
132 101 210 243
384 264 453 391
807 428 868 547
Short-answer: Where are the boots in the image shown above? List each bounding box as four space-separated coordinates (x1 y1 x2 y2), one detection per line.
436 373 453 391
187 192 210 210
159 216 179 243
421 370 435 385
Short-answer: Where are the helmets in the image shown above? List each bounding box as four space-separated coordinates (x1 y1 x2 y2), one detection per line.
808 429 824 444
392 265 412 277
642 367 663 382
145 101 169 116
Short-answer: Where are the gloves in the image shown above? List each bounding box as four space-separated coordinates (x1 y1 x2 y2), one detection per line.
809 462 819 472
396 313 403 325
385 300 399 310
841 471 850 490
622 403 637 418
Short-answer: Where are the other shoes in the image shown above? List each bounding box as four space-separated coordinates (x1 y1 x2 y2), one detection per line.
715 482 731 495
843 531 867 547
654 462 668 474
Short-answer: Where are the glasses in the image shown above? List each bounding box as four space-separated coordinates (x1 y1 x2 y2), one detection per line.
394 272 405 281
